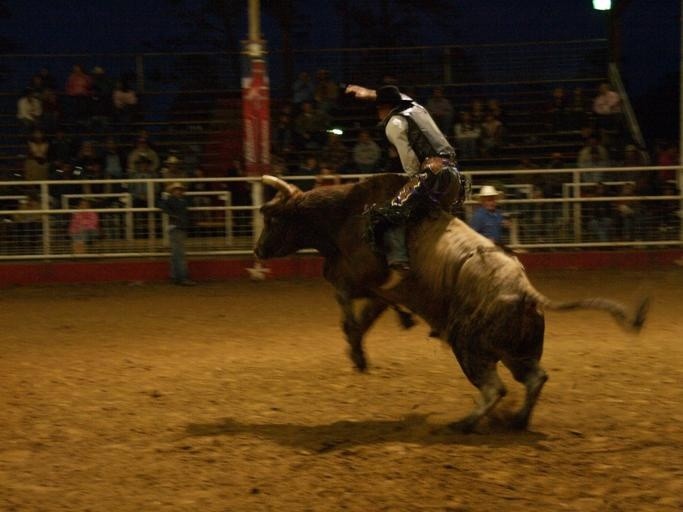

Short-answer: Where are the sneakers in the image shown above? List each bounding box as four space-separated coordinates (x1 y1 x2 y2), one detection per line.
380 264 410 290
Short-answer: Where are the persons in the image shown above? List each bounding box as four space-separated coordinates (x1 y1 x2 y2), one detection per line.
341 80 462 298
463 185 505 242
153 181 197 287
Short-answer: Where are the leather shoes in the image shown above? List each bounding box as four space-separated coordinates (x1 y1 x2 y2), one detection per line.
178 279 196 286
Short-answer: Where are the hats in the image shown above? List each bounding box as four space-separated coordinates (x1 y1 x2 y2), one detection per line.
136 154 152 165
164 156 179 164
167 182 187 191
372 85 415 104
475 186 504 197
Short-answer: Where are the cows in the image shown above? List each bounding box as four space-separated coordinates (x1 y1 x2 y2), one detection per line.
252 174 651 435
